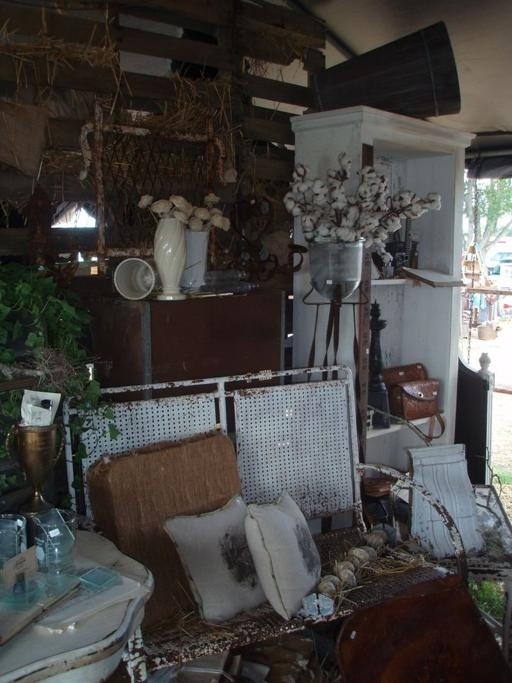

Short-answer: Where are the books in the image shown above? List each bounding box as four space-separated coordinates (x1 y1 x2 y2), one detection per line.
0 553 151 647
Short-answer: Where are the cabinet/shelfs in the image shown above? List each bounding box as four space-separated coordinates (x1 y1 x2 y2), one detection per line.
86 289 286 400
290 109 477 477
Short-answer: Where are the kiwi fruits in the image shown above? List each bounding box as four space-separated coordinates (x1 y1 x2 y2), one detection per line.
318 521 396 597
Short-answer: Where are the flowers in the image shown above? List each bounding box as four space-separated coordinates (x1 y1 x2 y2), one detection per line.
138 193 194 225
189 193 232 233
285 155 443 267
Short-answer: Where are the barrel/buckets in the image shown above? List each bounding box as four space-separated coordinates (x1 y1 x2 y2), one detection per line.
0 512 26 561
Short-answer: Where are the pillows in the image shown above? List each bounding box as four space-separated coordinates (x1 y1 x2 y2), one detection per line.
244 492 324 624
163 495 267 627
86 432 240 629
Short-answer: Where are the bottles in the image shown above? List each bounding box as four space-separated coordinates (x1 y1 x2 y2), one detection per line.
207 269 261 296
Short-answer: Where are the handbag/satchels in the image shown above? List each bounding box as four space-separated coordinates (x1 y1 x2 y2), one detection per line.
383 362 426 392
391 378 441 419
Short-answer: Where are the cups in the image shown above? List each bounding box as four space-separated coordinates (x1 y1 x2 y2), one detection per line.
1 512 26 596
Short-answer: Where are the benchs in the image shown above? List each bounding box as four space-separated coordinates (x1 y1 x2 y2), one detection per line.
62 365 469 683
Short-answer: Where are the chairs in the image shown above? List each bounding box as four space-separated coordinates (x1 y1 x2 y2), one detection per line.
334 578 512 683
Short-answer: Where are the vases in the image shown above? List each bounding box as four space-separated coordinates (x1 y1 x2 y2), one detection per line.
153 219 188 301
307 237 364 299
114 257 157 300
182 228 209 294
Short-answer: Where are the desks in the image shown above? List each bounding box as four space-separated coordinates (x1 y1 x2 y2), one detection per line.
0 530 156 683
